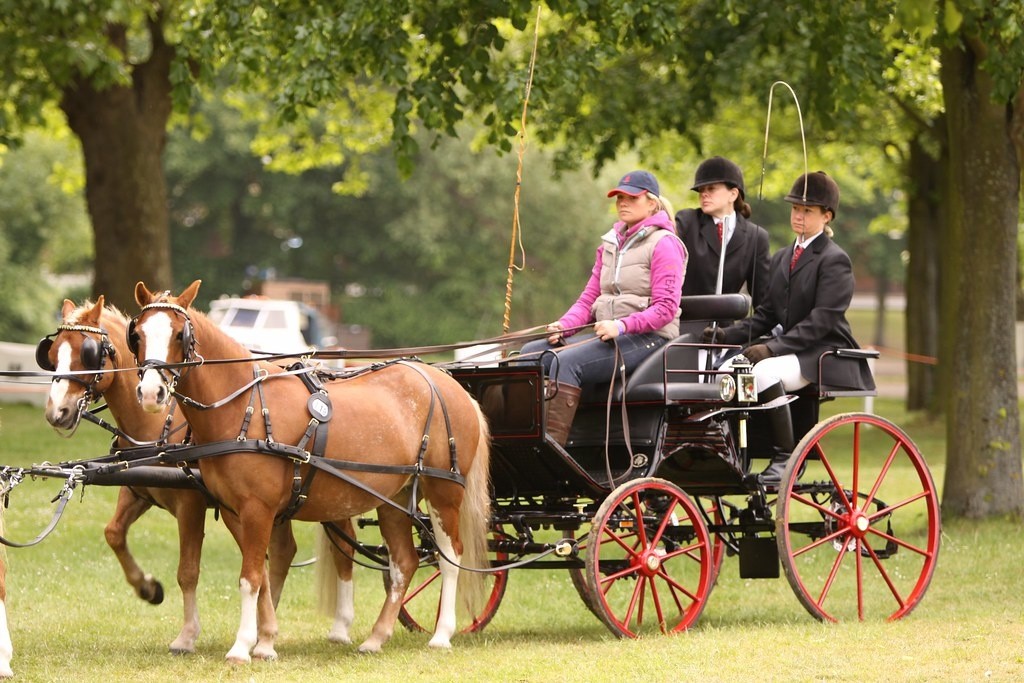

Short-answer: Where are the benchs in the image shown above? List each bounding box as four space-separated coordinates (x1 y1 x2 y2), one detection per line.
790 348 881 448
578 292 751 403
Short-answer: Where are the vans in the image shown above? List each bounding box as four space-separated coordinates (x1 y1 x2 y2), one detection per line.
207 298 326 360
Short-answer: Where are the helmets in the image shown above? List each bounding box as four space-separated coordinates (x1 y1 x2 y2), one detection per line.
690 155 745 201
784 171 839 221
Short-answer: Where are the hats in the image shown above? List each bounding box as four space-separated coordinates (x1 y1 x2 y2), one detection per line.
607 169 660 198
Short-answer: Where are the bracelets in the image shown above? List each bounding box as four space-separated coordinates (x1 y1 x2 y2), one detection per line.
614 318 624 337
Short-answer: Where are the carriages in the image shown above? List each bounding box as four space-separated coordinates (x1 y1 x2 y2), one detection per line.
0 277 943 660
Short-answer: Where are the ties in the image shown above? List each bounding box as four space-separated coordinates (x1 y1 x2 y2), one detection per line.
717 223 722 246
790 246 804 271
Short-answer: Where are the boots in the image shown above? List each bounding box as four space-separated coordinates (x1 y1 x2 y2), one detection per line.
758 380 807 486
544 380 582 448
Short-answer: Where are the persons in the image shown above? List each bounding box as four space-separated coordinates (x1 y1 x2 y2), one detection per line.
675 156 774 340
516 170 687 450
702 170 861 485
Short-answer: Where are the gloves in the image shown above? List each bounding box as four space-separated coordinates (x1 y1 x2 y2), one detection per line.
702 327 724 345
742 344 773 366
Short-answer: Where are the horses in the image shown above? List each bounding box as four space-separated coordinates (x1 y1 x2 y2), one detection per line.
45 295 357 657
133 279 494 666
